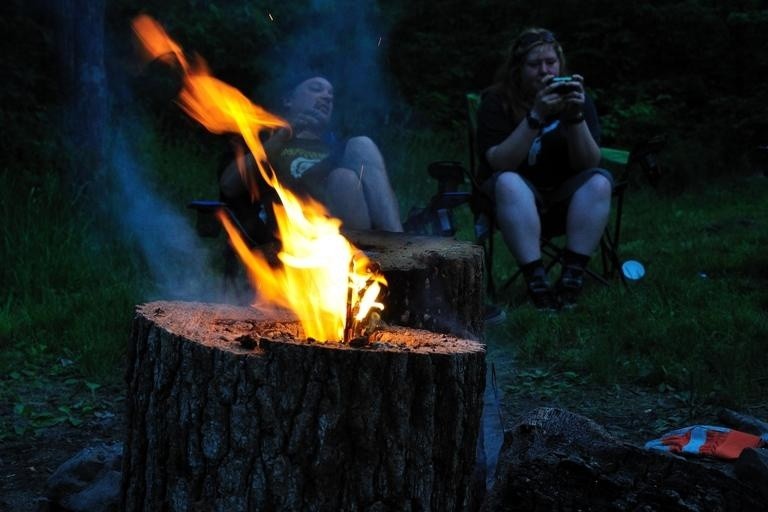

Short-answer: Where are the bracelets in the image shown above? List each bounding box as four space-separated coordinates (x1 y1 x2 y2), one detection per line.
567 115 586 124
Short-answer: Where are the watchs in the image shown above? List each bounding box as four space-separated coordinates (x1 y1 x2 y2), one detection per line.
526 111 544 131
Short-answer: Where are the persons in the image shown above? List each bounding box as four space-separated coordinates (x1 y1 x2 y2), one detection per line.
478 27 614 310
218 69 405 270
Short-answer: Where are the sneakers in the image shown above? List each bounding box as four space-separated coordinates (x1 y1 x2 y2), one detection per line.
486 304 506 327
525 275 553 309
556 263 585 302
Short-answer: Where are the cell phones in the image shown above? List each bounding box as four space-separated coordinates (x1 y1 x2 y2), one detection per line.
548 77 575 94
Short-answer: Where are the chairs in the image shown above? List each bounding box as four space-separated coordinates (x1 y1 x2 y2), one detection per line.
187 150 249 283
431 93 632 303
